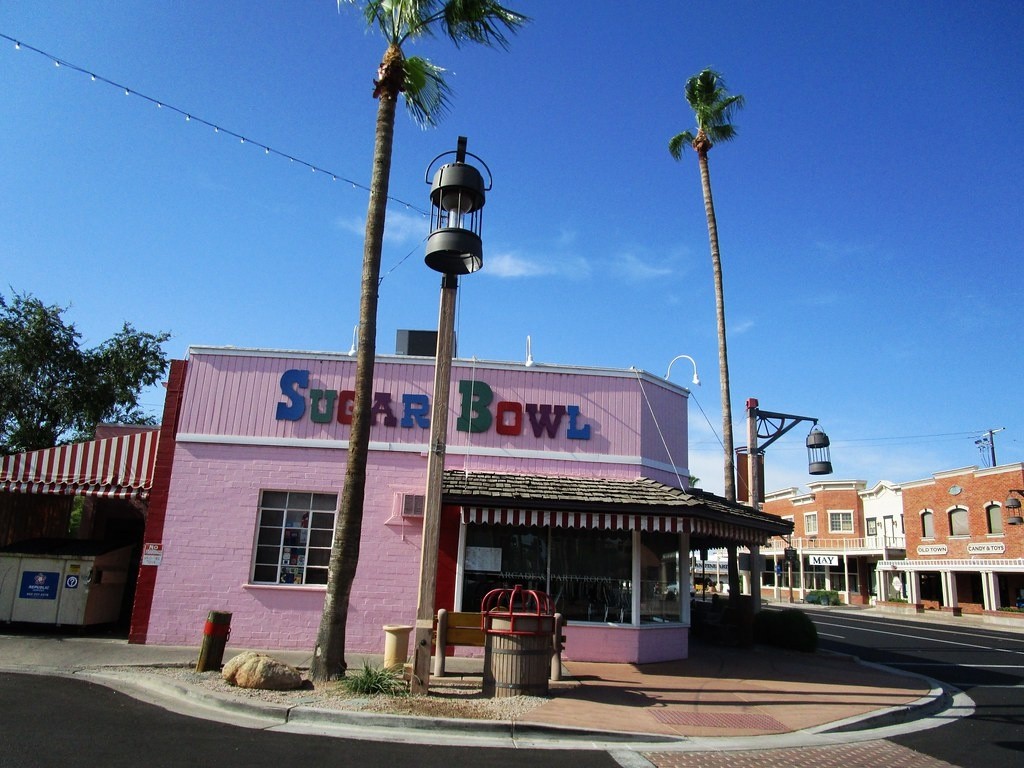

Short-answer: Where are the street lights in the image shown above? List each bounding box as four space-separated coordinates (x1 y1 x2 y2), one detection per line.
523 335 536 368
348 324 360 358
665 354 702 387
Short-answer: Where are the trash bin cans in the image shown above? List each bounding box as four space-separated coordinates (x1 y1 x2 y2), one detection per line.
0 538 128 635
